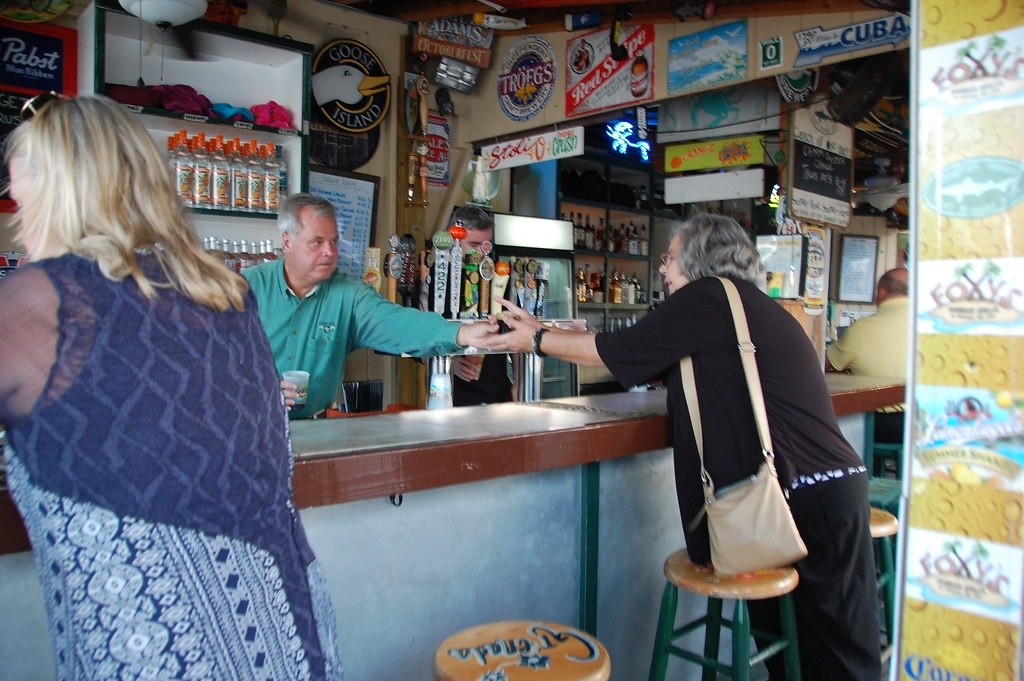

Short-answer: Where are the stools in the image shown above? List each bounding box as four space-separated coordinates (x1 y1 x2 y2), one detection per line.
435 621 610 681
647 549 802 681
865 444 903 662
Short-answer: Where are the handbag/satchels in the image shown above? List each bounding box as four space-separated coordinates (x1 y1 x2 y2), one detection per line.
702 457 808 577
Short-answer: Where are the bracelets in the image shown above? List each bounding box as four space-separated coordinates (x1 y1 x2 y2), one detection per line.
532 328 547 359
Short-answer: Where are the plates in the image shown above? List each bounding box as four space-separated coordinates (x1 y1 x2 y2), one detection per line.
570 42 596 74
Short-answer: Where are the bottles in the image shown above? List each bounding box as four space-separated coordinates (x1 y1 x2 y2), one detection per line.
198 236 283 273
609 266 647 304
628 185 649 209
573 263 605 304
610 310 638 333
630 50 649 98
609 220 650 256
561 211 606 251
168 129 287 215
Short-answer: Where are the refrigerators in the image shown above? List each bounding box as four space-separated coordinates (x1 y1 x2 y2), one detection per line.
492 211 579 401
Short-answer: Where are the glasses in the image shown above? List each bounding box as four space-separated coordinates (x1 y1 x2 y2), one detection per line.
20 92 60 121
659 252 676 265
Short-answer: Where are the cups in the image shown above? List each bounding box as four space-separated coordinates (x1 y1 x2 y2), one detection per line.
282 370 310 405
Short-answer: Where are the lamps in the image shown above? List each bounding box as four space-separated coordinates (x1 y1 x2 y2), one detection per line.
118 1 208 32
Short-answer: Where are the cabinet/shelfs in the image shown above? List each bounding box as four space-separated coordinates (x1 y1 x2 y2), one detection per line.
510 150 757 397
76 1 310 276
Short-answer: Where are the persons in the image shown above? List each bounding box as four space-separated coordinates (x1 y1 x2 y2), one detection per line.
1 93 344 681
823 267 908 478
421 205 525 406
233 190 513 422
471 215 885 680
